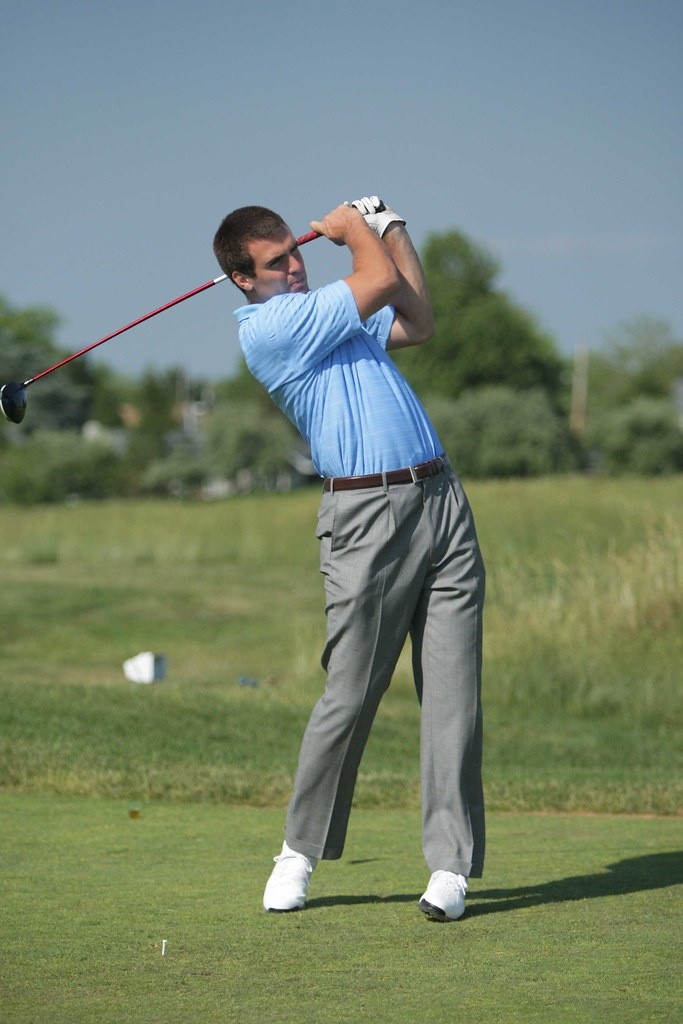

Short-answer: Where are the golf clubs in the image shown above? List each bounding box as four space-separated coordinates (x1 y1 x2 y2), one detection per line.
0 197 387 424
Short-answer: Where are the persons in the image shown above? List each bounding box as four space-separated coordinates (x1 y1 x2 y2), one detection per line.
214 196 486 921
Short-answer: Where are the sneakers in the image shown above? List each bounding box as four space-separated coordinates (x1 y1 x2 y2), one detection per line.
262 840 319 912
417 870 468 923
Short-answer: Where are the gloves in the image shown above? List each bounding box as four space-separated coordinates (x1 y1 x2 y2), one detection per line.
342 195 407 240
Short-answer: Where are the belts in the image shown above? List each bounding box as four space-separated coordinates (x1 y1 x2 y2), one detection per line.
322 451 450 493
